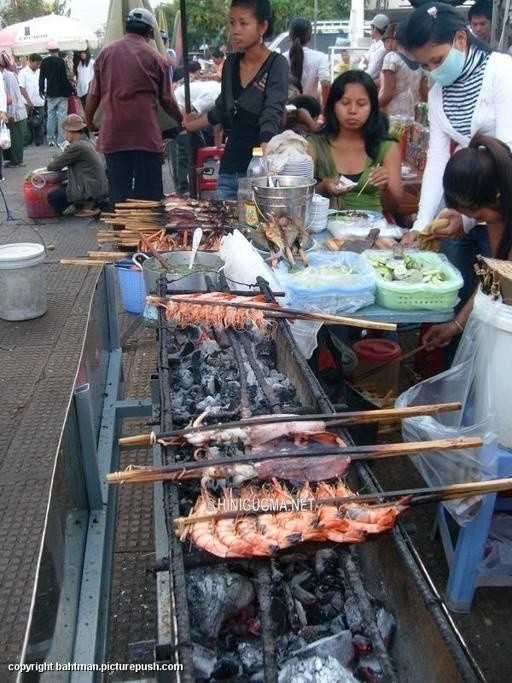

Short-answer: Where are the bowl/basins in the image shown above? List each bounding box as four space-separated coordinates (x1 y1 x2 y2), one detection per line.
32 166 70 184
249 175 318 251
329 210 383 239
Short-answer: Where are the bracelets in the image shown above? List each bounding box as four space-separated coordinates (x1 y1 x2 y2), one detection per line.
452 318 463 330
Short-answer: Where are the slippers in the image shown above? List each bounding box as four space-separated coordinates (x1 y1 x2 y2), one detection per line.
74 207 102 218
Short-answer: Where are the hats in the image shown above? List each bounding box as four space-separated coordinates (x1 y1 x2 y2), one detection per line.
380 24 398 40
128 8 156 29
369 12 389 31
61 113 87 132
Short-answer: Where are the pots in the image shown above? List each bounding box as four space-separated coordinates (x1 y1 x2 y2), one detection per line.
131 250 227 301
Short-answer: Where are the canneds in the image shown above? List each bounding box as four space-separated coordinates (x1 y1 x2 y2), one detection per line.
388 101 430 171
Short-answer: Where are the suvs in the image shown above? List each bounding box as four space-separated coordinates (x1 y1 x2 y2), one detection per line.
267 20 374 56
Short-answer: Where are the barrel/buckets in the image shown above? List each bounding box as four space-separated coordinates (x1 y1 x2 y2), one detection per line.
468 282 512 453
114 251 148 314
249 173 317 234
0 217 48 322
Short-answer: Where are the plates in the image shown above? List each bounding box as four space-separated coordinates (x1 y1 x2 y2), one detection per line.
400 163 418 181
251 237 317 260
277 153 329 234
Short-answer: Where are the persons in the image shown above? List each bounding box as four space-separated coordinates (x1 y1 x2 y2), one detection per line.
0 2 512 317
421 127 512 352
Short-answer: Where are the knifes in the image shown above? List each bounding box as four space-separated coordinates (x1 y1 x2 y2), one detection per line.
337 228 381 255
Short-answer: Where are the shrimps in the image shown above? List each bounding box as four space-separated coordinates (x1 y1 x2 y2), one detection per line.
189 472 414 558
164 289 279 334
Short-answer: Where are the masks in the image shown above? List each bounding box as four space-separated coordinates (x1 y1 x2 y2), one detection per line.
420 49 467 87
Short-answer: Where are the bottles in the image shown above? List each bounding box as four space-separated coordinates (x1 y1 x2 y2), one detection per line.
236 148 270 228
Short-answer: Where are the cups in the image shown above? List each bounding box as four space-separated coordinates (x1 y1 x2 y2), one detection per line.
351 338 399 401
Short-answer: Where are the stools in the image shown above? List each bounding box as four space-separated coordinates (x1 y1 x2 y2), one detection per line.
432 445 512 614
197 146 224 190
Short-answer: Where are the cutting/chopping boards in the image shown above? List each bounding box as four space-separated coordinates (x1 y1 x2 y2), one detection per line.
328 237 396 253
